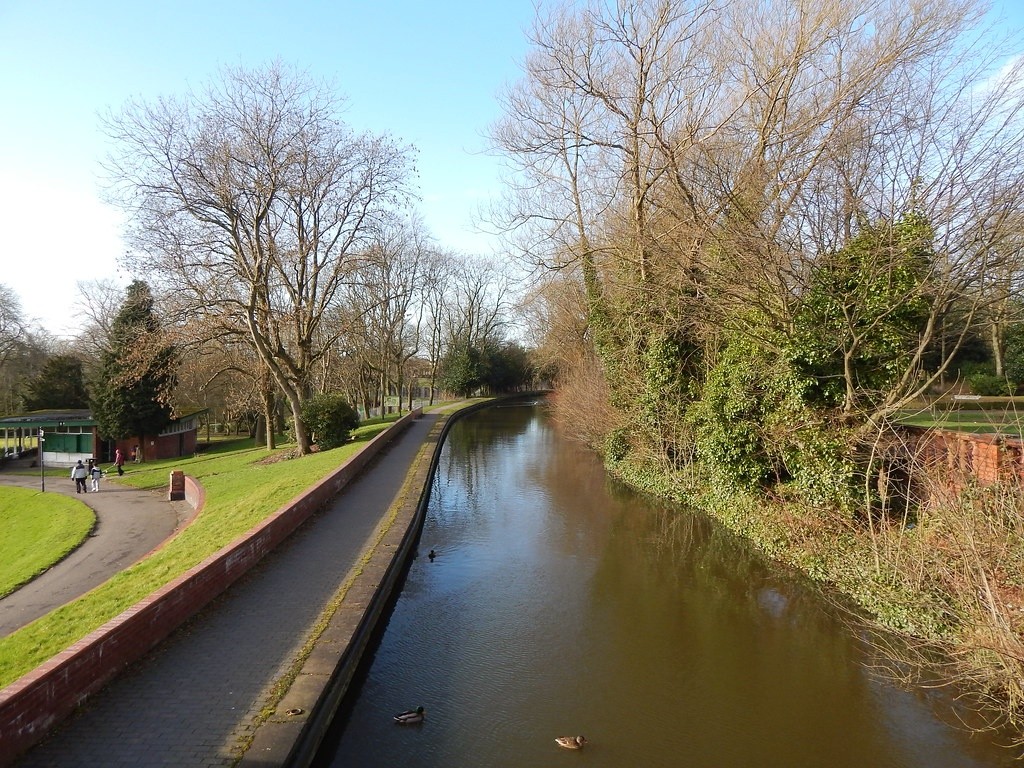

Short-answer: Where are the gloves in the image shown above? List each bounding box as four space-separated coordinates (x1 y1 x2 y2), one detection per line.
85 476 87 478
72 478 74 481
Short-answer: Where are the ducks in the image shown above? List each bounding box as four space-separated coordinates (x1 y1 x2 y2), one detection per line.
554 735 589 749
394 706 427 723
428 550 436 559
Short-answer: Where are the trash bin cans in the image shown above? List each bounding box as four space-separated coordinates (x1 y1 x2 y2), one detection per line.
169 470 185 501
85 459 97 474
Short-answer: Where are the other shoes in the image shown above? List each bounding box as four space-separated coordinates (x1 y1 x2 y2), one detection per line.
76 491 80 494
92 489 96 491
96 490 98 493
84 489 87 493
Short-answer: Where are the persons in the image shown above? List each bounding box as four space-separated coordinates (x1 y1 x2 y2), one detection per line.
71 460 87 494
90 463 102 492
114 449 124 476
